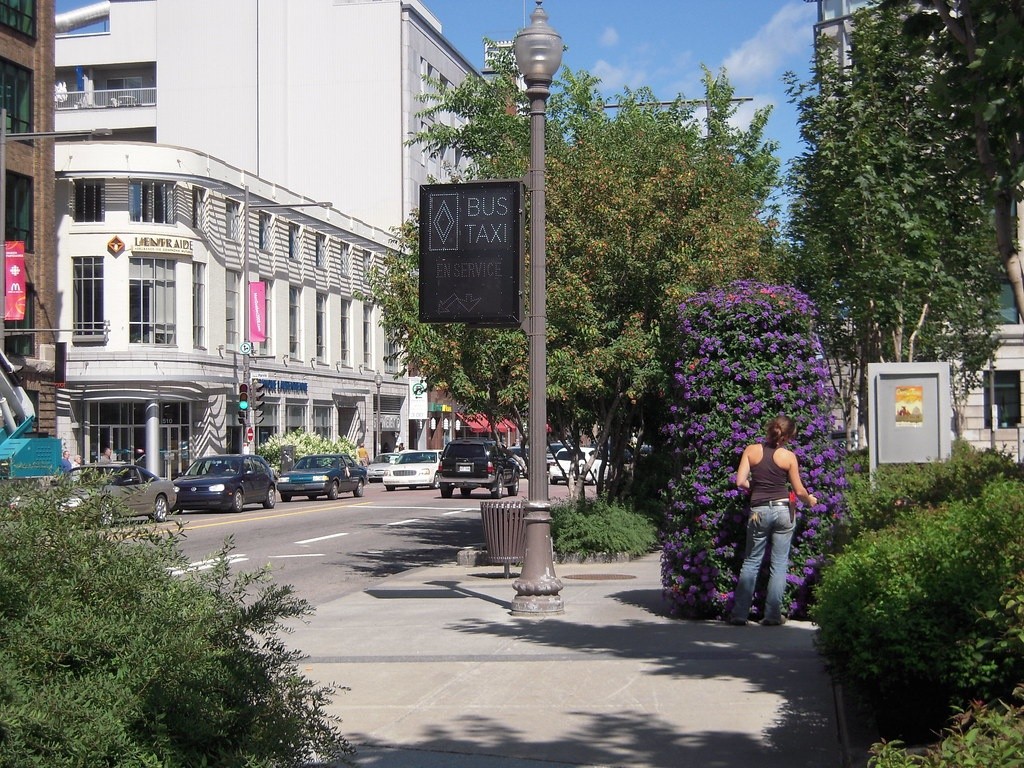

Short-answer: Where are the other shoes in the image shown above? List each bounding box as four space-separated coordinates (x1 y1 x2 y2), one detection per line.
763 615 786 626
730 616 748 625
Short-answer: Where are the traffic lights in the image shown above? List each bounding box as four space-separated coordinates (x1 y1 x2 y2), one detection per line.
238 383 248 411
252 383 264 410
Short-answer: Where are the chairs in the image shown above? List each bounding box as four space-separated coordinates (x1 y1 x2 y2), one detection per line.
310 464 323 468
213 463 230 473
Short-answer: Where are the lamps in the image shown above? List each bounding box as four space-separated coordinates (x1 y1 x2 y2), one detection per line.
335 359 341 372
444 417 448 430
250 349 259 363
358 364 363 374
215 344 224 359
310 357 316 371
456 419 460 431
85 362 89 371
154 361 158 369
430 418 437 430
418 420 423 430
281 354 289 367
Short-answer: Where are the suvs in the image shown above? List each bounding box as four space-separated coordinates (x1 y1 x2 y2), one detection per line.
438 437 520 500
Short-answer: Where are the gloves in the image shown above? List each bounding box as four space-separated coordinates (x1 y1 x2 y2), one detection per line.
747 511 763 530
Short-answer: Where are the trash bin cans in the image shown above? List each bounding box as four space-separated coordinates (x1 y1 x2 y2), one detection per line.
480 501 531 564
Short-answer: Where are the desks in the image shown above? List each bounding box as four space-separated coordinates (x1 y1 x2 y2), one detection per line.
118 95 135 107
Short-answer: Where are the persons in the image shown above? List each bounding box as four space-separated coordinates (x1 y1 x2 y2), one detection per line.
62 451 81 481
357 443 368 467
100 448 112 474
330 460 349 470
308 458 320 469
725 416 817 626
399 443 404 452
225 460 239 473
205 464 216 475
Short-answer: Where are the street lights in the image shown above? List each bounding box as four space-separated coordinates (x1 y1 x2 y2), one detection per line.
0 109 114 355
242 184 334 456
374 370 383 454
509 1 568 618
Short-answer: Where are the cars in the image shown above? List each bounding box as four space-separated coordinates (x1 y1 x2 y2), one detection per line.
549 446 610 485
383 449 445 491
172 455 277 513
367 452 399 483
507 443 566 478
10 464 177 529
276 454 367 502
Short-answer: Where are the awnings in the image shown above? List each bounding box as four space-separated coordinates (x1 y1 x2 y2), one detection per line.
455 412 517 433
